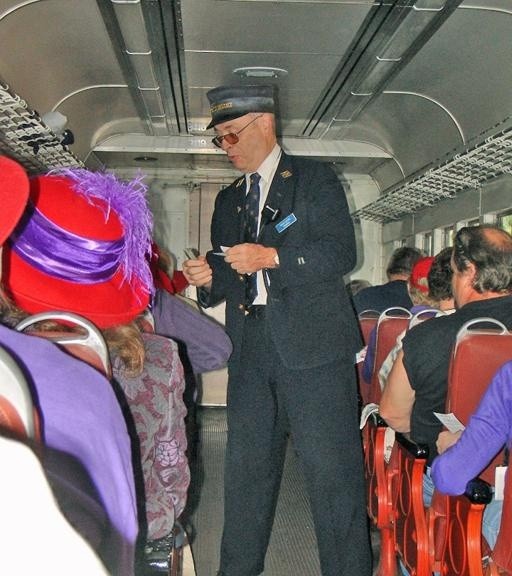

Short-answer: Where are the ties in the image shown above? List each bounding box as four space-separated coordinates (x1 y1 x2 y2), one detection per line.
239 174 261 309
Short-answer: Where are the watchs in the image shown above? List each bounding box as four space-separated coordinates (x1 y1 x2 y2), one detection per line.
273 254 280 268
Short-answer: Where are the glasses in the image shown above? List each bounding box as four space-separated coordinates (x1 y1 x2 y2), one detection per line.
212 115 265 149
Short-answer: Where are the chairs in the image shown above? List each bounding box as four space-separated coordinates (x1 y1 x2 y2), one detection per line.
2 307 190 576
357 307 512 576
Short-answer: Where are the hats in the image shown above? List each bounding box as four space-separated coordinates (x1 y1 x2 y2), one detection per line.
0 152 153 329
410 256 436 290
147 240 188 296
204 82 274 129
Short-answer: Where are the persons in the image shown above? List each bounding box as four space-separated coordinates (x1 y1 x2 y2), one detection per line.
348 223 510 551
1 288 234 576
183 84 372 575
